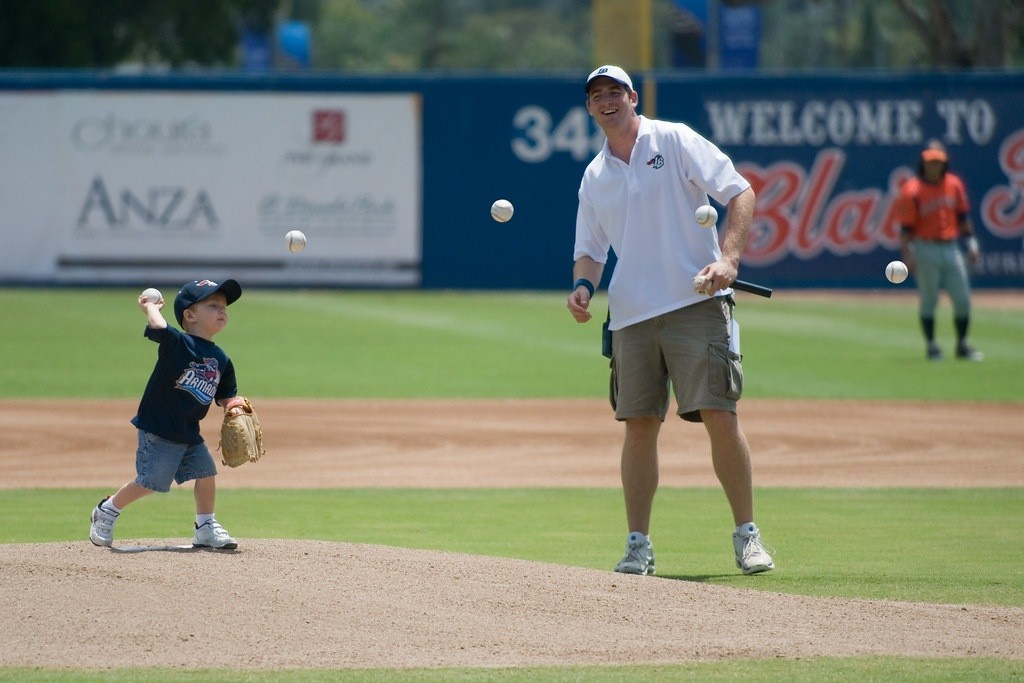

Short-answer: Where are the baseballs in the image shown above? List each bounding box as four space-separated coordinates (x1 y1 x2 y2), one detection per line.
140 287 162 305
490 198 514 223
694 203 718 229
884 260 909 284
284 230 307 253
693 274 713 296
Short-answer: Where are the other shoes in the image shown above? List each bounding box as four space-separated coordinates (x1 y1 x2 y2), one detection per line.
956 347 982 361
927 346 941 359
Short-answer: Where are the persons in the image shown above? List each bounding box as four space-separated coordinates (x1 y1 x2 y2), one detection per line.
899 139 984 361
567 65 775 575
90 279 246 548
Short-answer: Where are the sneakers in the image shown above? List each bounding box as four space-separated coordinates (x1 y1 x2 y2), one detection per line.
614 530 656 576
732 521 777 576
193 520 238 549
89 497 120 547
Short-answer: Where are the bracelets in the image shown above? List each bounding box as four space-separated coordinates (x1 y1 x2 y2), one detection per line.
574 278 595 298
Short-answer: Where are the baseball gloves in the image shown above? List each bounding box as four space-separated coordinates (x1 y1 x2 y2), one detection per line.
215 398 265 468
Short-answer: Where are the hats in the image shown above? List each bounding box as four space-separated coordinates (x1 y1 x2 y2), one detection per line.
584 66 633 93
174 278 242 329
920 139 948 162
